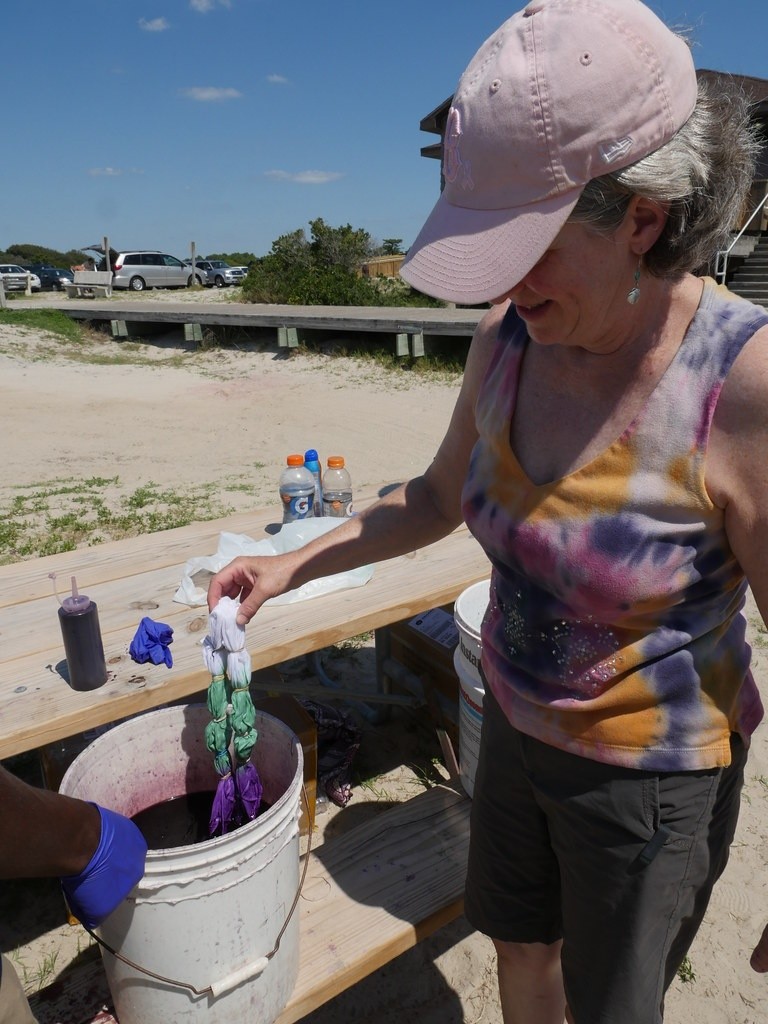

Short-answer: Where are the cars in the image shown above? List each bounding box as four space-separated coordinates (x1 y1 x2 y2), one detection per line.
0 263 42 292
21 265 74 291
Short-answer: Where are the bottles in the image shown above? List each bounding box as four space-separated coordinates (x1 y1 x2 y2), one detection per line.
279 455 315 523
304 449 321 510
49 571 108 691
322 456 353 518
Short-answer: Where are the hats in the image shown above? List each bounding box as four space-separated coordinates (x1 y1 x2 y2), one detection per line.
397 0 699 306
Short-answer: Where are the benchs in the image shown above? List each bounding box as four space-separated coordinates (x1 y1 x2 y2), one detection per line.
3 762 480 1024
62 271 113 299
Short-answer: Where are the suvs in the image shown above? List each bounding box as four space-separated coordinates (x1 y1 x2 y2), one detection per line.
186 259 244 288
233 266 248 281
80 243 209 292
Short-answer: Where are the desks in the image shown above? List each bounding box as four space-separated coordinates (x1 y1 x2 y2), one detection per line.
0 474 496 760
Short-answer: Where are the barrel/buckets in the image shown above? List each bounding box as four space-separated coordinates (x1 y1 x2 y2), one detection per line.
452 579 489 798
56 702 303 1023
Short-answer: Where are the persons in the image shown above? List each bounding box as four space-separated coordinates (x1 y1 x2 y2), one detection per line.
0 764 148 1024
82 258 94 292
204 0 768 1024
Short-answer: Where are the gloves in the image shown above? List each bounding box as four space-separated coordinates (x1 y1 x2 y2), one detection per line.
61 801 148 930
